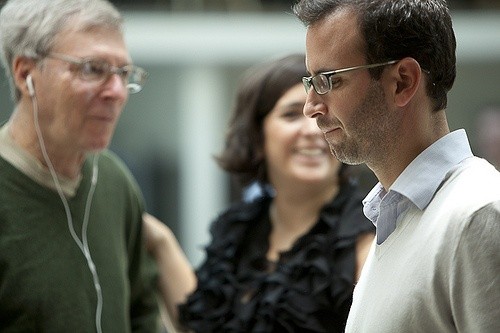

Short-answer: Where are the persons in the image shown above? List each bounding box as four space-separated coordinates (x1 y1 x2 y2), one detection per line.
0 0 169 332
288 1 500 333
142 51 379 333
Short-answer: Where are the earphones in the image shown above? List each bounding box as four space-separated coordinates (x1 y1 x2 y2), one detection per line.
25 75 35 97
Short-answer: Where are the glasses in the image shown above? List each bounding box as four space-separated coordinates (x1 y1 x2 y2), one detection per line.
40 51 146 94
302 59 431 96
